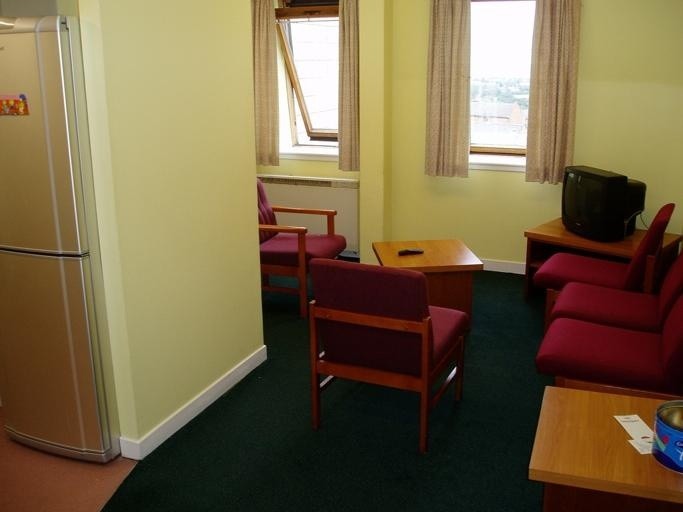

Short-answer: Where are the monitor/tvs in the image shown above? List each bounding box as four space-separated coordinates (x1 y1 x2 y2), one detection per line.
561 165 646 244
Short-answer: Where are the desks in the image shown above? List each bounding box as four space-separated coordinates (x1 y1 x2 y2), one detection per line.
526 385 681 512
523 216 683 307
372 238 486 319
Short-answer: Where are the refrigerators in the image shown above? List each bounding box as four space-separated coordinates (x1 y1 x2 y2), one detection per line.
0 12 123 467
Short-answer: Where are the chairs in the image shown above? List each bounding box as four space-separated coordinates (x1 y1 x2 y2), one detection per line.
532 202 682 402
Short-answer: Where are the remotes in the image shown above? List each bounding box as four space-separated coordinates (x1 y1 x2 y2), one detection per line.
398 249 423 255
